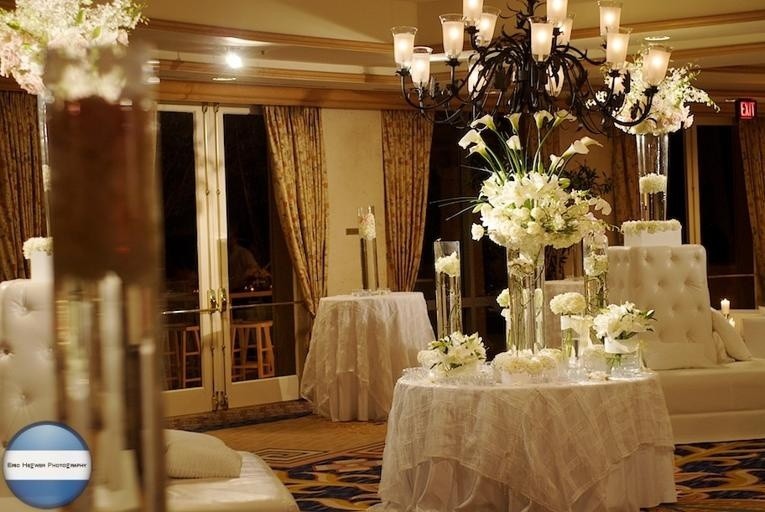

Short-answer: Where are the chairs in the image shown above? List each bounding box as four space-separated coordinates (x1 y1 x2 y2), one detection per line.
1 278 59 446
1 444 302 512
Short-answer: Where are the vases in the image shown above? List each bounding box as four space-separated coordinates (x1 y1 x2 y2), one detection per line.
29 254 55 280
623 233 683 248
636 131 668 220
356 204 375 291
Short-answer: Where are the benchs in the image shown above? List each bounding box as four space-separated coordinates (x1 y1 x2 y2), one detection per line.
541 246 765 443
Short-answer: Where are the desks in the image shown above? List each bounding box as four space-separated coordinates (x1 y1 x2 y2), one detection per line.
375 364 678 510
298 289 438 425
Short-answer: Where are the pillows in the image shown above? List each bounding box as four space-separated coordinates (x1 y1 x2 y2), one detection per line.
163 430 244 479
708 330 737 364
710 308 752 364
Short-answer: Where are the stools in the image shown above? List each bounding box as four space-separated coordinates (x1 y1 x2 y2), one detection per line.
167 319 278 388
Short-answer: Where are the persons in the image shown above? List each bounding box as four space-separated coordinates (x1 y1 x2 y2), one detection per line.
229 225 258 291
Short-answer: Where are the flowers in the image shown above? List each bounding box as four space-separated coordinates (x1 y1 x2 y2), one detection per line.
359 215 375 240
619 218 683 235
413 222 659 385
639 173 666 195
439 101 619 274
589 44 722 136
0 0 150 104
22 235 54 259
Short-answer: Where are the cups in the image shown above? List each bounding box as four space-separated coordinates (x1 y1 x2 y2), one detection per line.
720 301 730 320
401 317 647 387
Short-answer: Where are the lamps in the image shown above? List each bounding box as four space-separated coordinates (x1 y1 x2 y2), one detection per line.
388 0 670 137
